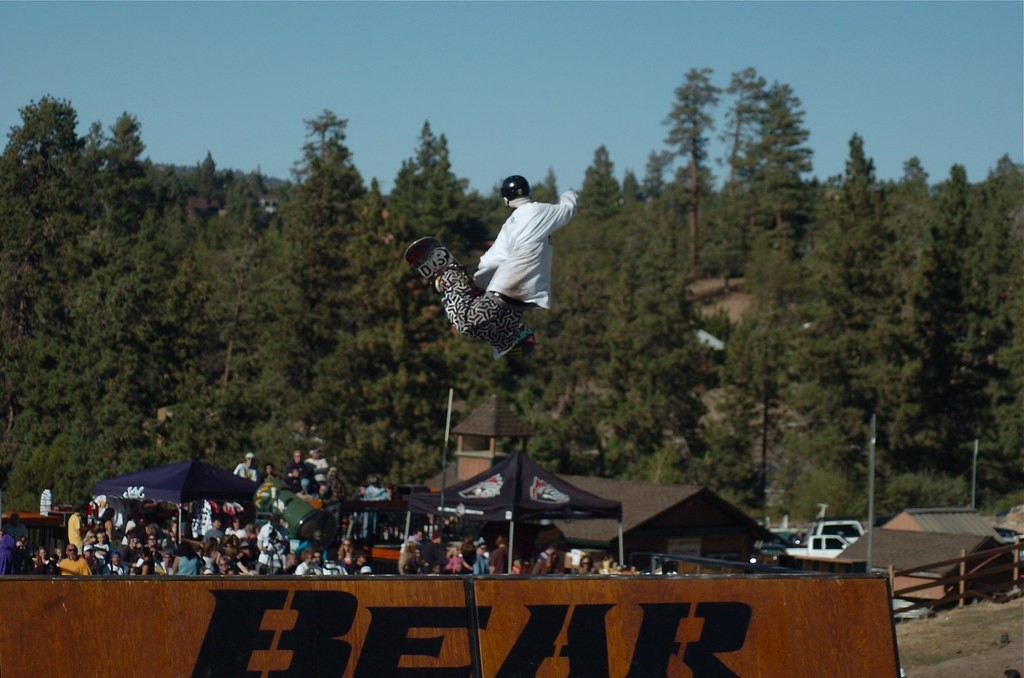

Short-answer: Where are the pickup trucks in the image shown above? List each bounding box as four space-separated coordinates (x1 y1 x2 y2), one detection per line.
785 534 850 559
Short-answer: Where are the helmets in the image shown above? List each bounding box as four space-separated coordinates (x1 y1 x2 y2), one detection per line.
500 175 531 207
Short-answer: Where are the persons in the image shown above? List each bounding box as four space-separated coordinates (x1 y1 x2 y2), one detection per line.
579 553 639 574
0 497 373 575
399 528 515 574
793 531 803 546
531 540 566 574
353 476 400 542
414 175 579 359
260 462 279 484
233 453 258 482
285 449 332 500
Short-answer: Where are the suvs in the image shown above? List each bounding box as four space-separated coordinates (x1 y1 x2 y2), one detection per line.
804 519 864 546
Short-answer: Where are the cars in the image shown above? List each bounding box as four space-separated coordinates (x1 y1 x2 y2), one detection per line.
769 528 808 546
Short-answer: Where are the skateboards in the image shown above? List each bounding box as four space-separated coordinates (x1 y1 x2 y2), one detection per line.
403 236 536 363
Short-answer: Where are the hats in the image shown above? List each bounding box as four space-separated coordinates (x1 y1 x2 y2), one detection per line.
111 551 120 557
84 545 93 552
245 452 255 459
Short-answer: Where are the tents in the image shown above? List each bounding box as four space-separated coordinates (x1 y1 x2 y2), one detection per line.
403 450 623 574
89 457 259 545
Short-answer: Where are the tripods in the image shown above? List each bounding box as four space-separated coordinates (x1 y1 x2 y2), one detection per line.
264 539 284 576
